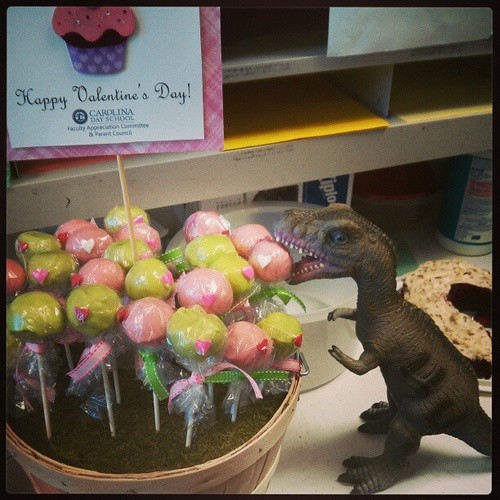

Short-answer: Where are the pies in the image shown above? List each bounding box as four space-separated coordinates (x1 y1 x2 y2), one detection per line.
399 259 492 364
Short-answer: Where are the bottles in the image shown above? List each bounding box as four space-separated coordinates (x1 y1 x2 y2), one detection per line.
434 149 492 255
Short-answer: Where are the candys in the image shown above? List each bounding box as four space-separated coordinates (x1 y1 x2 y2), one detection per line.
6 208 303 450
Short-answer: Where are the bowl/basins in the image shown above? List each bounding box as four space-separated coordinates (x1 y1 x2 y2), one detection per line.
167 203 358 394
